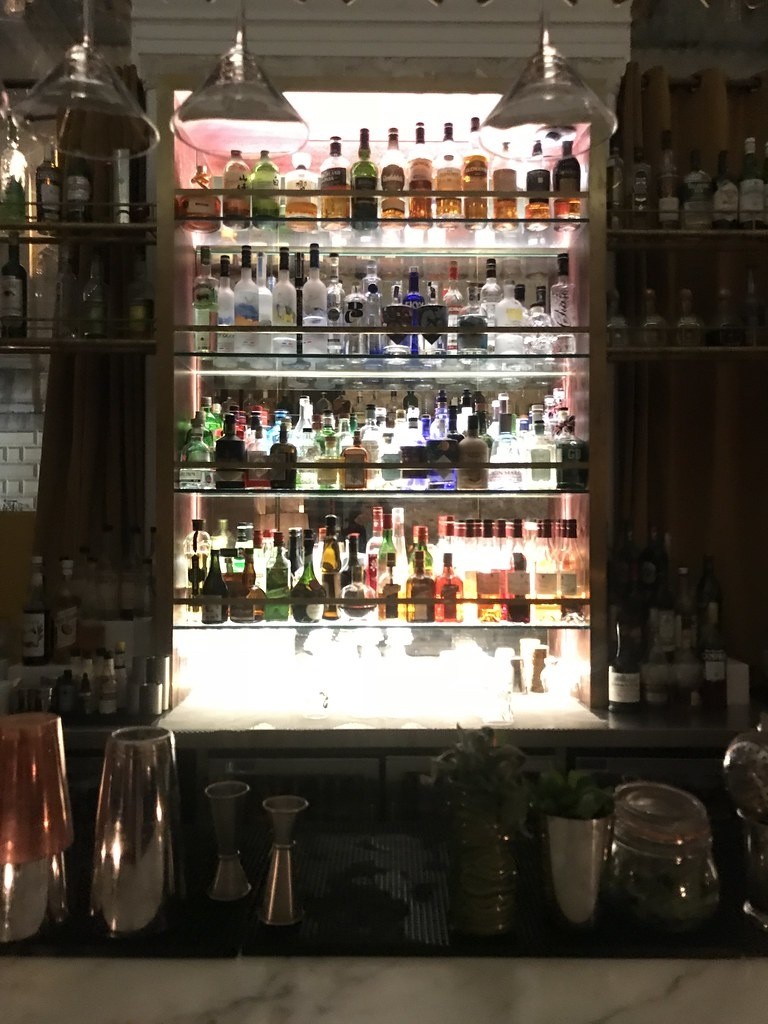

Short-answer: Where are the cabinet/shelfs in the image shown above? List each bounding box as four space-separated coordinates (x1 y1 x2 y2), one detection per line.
0 189 768 714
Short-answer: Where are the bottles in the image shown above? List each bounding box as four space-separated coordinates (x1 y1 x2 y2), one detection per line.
0 231 27 340
0 124 26 226
178 117 582 230
66 144 93 223
32 237 150 340
606 133 767 227
608 529 727 713
36 134 61 224
190 242 574 366
609 265 767 344
22 554 49 666
182 505 588 622
52 527 158 721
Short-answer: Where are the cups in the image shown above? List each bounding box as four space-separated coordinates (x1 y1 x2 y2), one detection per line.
178 384 587 491
89 726 182 941
140 683 162 715
